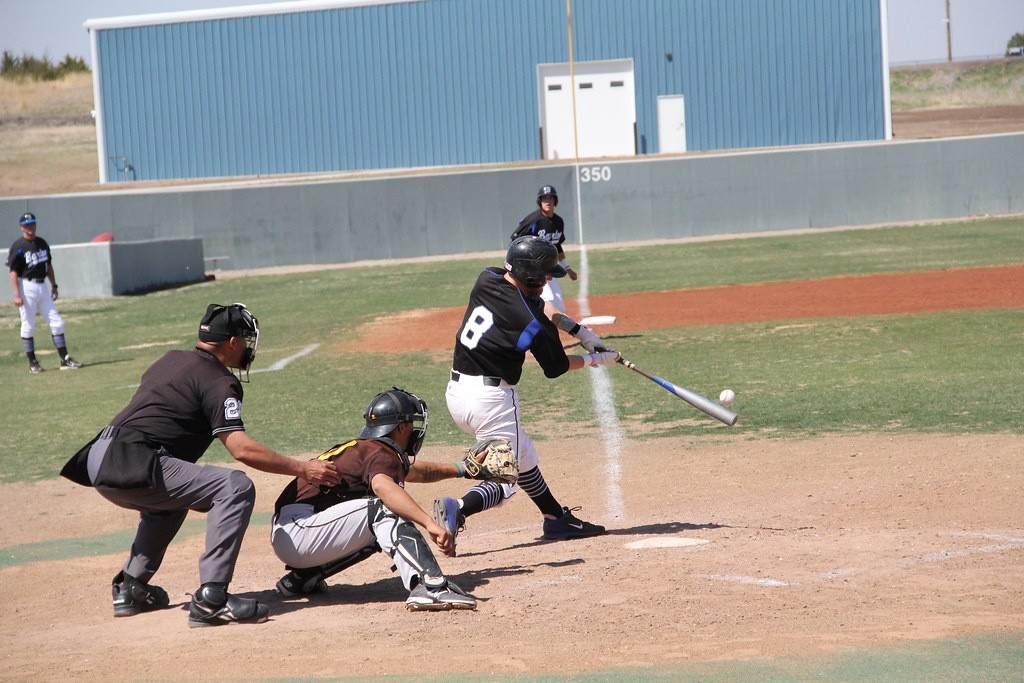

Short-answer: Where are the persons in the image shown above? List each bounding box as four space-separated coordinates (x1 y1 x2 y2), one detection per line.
4 212 83 372
509 185 577 312
271 386 518 610
59 303 341 627
433 235 618 557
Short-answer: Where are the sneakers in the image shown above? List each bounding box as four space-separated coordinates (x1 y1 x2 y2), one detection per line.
29 359 45 374
60 354 83 370
405 578 477 613
543 506 606 540
432 496 467 557
275 573 328 599
112 570 170 617
185 588 270 628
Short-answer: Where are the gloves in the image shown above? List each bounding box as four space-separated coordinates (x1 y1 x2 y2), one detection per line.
574 325 618 370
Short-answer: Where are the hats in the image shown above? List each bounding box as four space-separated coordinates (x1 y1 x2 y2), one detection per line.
198 303 259 342
18 212 37 225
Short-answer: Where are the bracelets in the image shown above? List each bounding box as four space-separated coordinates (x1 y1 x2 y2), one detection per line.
53 284 58 288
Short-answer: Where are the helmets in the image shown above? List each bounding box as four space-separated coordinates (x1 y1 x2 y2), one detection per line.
358 387 429 439
537 184 558 207
504 235 567 288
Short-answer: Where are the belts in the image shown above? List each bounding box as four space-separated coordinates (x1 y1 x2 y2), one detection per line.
451 371 501 388
26 278 45 283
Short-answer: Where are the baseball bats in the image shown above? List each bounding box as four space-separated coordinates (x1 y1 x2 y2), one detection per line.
579 342 739 428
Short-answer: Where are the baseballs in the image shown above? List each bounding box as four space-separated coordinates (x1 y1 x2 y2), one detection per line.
719 389 736 405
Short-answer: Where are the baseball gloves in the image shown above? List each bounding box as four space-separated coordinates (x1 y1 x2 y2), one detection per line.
462 437 519 485
